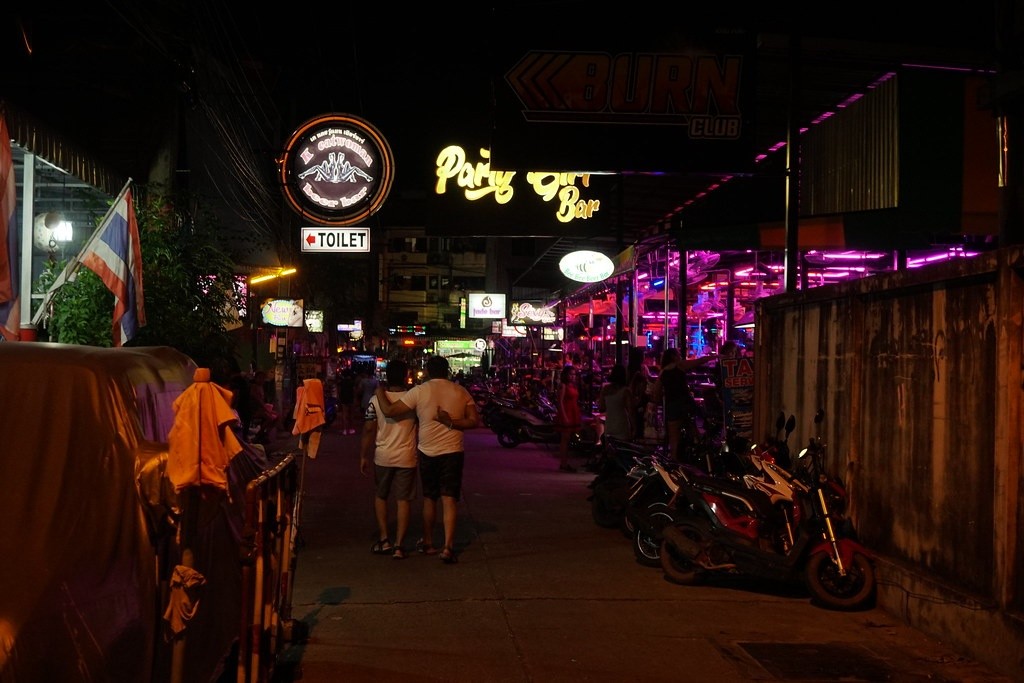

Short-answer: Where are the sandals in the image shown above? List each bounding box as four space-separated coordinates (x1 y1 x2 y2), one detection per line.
438 544 453 560
393 546 404 559
416 538 437 553
369 538 392 554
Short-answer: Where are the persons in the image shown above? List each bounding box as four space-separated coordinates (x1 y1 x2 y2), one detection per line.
557 366 580 472
372 357 483 560
357 361 421 559
338 366 378 430
600 341 752 468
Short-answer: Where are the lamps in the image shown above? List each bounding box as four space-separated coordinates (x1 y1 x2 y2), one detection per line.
32 180 68 255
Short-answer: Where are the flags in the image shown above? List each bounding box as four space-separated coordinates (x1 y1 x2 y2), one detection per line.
0 110 22 341
79 185 148 347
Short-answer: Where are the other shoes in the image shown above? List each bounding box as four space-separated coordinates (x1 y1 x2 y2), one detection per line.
558 463 577 472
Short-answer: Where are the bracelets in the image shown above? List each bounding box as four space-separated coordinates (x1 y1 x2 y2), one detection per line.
448 420 454 431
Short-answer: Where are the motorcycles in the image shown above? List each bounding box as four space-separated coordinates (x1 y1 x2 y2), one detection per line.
244 326 884 611
